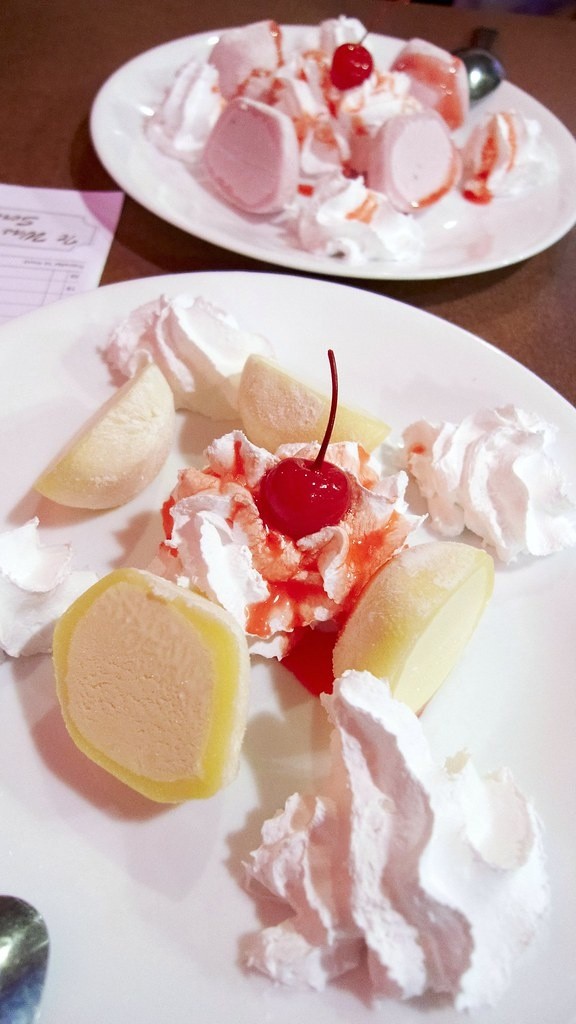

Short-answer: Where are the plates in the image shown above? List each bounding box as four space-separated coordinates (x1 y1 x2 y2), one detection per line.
83 25 576 282
0 269 576 1024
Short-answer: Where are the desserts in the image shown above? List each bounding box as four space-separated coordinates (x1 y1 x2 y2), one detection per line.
147 12 537 255
2 293 576 1016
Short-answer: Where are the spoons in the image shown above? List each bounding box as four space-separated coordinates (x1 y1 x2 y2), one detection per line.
0 895 51 1024
450 28 505 106
